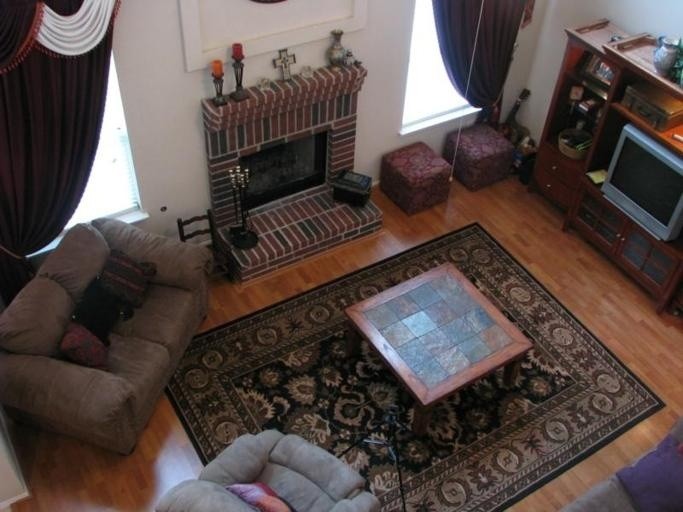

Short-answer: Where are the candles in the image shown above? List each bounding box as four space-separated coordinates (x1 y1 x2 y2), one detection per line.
211 44 242 78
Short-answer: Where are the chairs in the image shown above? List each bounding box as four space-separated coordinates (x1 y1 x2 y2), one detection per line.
155 428 384 512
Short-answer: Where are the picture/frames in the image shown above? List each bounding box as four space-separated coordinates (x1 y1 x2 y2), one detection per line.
178 1 368 74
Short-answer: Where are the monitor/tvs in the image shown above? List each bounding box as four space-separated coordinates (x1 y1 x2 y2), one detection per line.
601 124 682 242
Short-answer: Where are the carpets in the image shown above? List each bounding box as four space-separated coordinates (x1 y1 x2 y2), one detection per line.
164 221 667 511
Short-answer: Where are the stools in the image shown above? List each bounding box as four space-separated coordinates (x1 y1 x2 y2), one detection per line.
379 122 517 217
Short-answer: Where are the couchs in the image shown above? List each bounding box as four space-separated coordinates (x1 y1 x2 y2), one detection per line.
0 218 216 457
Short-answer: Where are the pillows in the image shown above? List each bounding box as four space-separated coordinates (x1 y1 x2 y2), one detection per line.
615 434 682 511
59 250 157 370
226 482 297 512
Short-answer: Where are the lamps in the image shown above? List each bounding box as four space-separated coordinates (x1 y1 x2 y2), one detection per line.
228 166 257 248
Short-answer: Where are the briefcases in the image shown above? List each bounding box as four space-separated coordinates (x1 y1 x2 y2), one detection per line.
620 85 683 132
